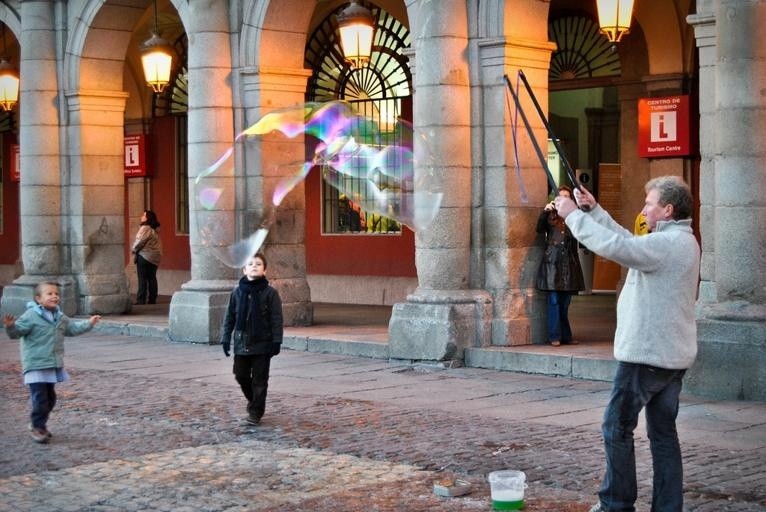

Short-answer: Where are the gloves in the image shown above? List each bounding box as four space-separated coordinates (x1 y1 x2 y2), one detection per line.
270 343 280 358
223 343 230 356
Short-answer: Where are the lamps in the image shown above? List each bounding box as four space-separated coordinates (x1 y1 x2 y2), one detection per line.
0 0 20 112
596 0 635 51
335 0 375 62
138 0 172 94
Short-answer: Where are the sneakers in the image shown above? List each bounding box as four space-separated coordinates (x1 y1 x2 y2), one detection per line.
570 340 579 344
588 500 635 512
28 423 52 443
247 402 260 426
552 341 560 346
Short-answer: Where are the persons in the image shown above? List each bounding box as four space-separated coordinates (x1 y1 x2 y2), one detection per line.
551 176 701 512
533 187 586 345
131 209 162 306
3 281 101 443
220 253 283 424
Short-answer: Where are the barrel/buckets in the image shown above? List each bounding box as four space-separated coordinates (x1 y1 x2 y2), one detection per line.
488 470 528 511
